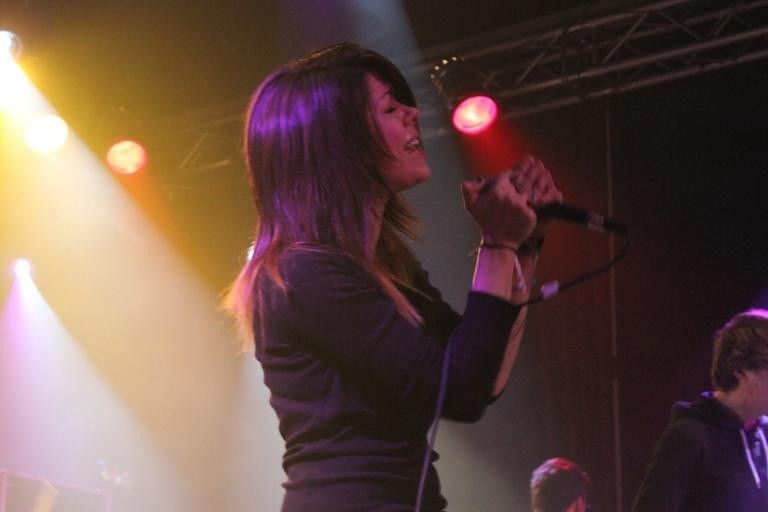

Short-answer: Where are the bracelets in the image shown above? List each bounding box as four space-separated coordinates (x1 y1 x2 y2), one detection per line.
472 238 527 261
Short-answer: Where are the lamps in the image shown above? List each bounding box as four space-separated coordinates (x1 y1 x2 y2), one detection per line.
100 108 148 176
429 53 500 136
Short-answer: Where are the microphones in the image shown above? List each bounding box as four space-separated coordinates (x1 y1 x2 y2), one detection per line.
479 177 629 242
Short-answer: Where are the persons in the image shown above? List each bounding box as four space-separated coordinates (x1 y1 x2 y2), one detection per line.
624 309 768 508
214 42 566 510
525 457 595 512
